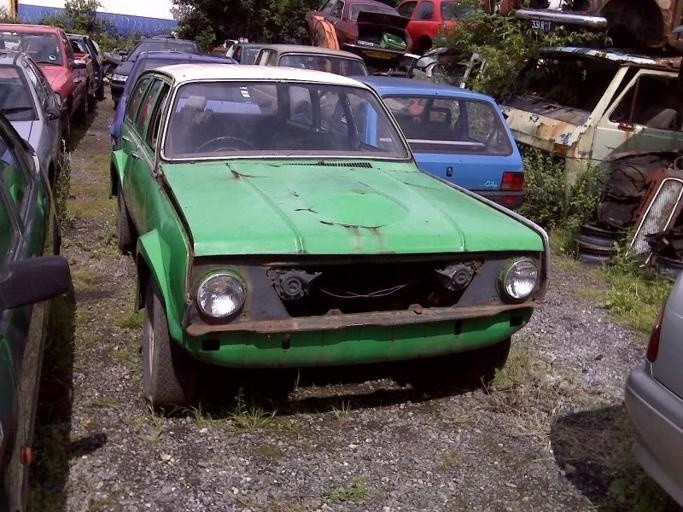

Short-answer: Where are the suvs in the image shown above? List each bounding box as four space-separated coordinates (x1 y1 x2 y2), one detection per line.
112 61 547 413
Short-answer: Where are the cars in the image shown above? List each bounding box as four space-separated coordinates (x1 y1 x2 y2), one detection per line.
112 49 262 161
618 267 682 510
0 114 69 512
0 24 106 194
297 74 527 213
490 45 683 253
110 35 202 113
220 1 682 120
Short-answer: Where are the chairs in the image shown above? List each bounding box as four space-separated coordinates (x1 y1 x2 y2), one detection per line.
407 106 452 140
